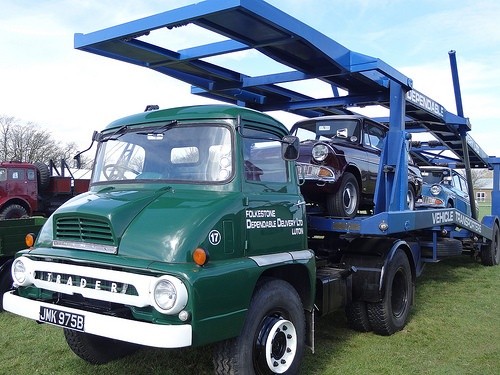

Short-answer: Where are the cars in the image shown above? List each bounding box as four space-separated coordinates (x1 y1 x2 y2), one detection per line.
415 167 480 220
288 115 424 219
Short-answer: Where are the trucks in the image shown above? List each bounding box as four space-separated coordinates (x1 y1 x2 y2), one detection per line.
2 0 500 375
0 157 93 220
